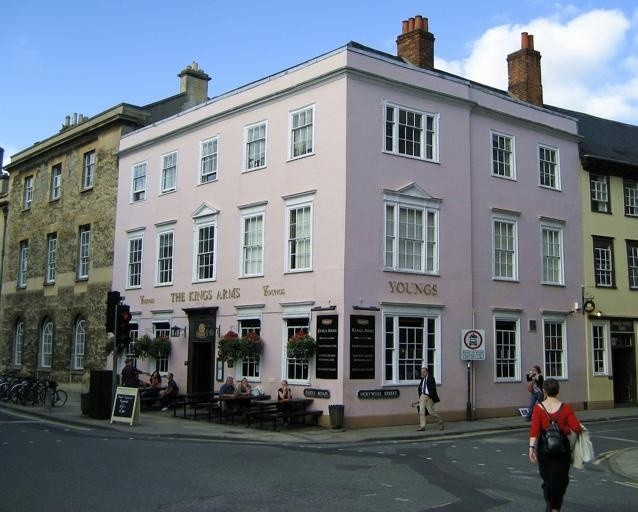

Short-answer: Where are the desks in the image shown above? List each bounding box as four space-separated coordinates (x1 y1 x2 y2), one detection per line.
221 394 270 431
136 386 166 411
174 392 218 423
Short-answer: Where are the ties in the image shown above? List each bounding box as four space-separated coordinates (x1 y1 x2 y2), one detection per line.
423 377 427 394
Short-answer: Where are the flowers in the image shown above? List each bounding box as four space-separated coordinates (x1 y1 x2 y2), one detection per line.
131 335 170 358
217 329 262 362
287 331 316 362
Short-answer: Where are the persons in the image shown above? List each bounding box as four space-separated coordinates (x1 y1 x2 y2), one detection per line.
525 364 544 421
121 360 150 388
220 377 238 425
416 367 445 431
237 378 251 395
160 373 178 411
528 378 582 512
276 380 292 427
141 370 161 398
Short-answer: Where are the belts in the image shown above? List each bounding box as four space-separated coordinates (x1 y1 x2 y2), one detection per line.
421 393 428 395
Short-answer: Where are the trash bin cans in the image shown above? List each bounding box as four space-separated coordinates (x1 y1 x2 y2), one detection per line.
80 393 90 415
328 405 345 432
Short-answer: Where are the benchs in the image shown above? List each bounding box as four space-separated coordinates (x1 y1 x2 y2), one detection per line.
171 398 324 431
140 394 187 411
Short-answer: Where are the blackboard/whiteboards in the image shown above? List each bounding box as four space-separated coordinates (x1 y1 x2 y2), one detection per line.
111 387 139 422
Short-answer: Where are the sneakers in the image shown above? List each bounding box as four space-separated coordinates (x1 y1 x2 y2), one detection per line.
162 407 168 411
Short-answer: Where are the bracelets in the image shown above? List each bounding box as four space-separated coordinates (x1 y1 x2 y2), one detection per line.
529 446 535 448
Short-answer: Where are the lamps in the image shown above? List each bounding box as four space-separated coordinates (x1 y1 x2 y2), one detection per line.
203 325 220 337
170 325 187 338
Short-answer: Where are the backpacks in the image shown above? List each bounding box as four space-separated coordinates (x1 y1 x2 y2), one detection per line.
538 401 565 456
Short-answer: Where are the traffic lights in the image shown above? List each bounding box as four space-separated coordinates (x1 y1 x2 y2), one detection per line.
105 289 133 346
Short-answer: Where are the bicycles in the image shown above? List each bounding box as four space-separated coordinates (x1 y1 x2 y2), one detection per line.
0 366 68 407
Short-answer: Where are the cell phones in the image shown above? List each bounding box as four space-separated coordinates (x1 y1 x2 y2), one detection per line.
529 370 532 373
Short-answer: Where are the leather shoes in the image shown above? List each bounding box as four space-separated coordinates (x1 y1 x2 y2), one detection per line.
416 427 424 431
439 422 444 430
